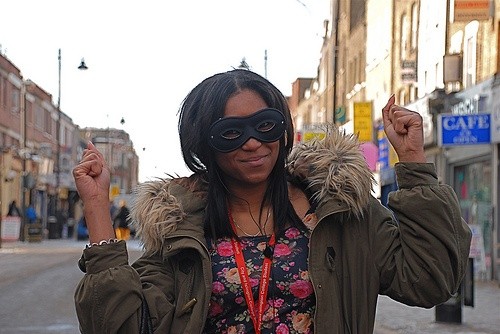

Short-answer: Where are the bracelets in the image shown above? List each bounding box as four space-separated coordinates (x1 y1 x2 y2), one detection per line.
84 238 119 249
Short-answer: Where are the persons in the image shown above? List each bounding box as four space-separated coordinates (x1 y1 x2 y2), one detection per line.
7 200 21 219
24 204 36 225
112 198 133 245
70 69 474 334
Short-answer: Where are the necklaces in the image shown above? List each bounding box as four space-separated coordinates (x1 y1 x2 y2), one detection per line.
230 188 275 238
